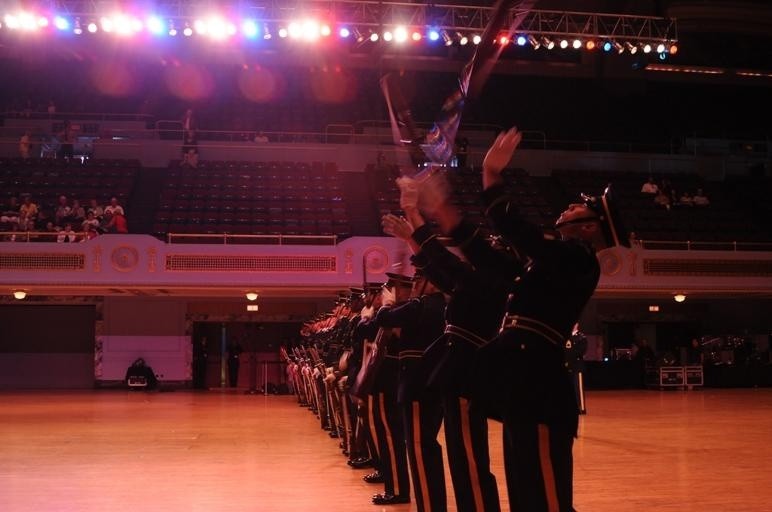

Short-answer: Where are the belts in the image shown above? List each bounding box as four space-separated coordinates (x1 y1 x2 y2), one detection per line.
397 348 428 359
322 352 328 357
328 343 343 349
501 313 569 348
341 346 354 352
443 322 490 351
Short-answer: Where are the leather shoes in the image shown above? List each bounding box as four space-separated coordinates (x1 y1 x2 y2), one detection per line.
299 401 410 506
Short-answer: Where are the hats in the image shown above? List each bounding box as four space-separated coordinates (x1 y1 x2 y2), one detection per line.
363 282 385 294
578 181 631 254
384 272 421 290
349 286 364 300
298 291 350 328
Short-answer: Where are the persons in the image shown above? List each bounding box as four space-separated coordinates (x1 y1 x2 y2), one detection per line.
634 338 656 386
15 128 38 159
0 192 128 244
251 128 271 143
194 334 209 387
279 122 630 511
637 174 714 211
691 337 706 369
180 104 199 142
225 334 243 386
18 96 38 117
45 98 61 119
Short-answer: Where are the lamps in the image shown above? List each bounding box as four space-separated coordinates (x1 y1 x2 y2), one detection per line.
0 15 679 55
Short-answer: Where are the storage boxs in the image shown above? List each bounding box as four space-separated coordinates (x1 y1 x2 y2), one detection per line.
645 366 704 388
